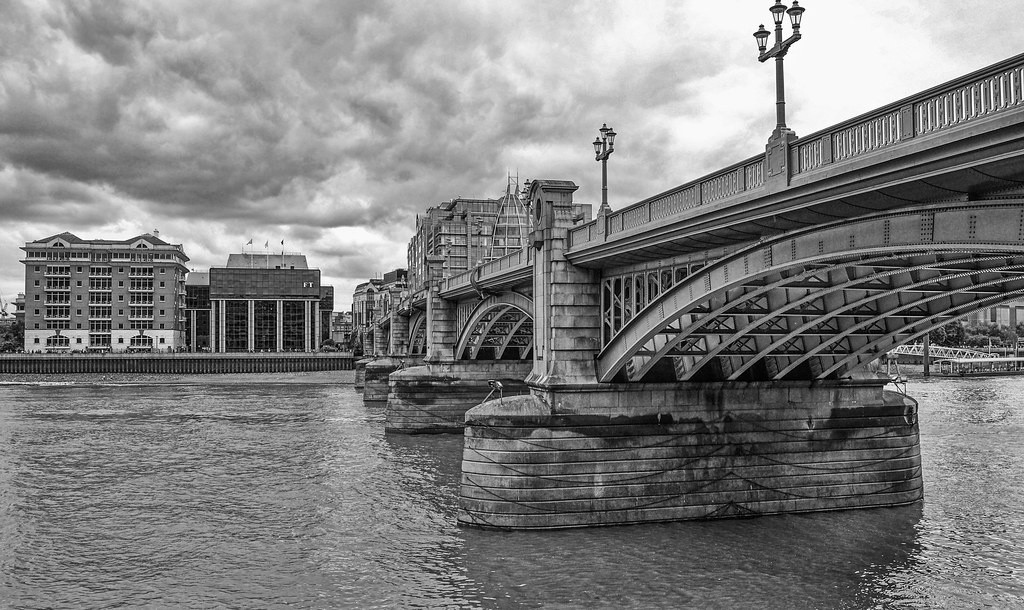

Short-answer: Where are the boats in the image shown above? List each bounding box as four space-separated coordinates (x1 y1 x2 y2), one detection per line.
927 353 1024 378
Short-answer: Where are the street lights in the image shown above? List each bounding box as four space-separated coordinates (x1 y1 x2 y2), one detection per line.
592 122 617 203
754 0 806 131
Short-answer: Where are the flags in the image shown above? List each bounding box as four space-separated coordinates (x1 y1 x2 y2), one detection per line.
264 241 269 249
280 240 284 245
246 238 253 245
